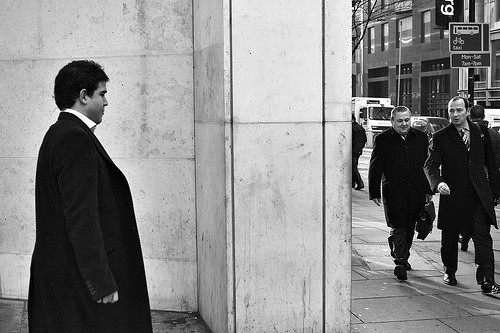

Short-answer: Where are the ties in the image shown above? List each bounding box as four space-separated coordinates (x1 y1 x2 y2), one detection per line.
461 129 470 151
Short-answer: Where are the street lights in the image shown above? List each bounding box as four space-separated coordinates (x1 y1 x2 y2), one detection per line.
375 16 403 106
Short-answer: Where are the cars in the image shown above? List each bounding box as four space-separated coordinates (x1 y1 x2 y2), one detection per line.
410 115 450 139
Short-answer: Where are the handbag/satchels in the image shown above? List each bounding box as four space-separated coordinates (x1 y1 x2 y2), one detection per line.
416 201 436 239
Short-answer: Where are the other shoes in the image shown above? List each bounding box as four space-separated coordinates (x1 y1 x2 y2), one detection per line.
459 235 468 251
355 185 364 190
405 262 411 270
352 183 355 188
394 265 408 281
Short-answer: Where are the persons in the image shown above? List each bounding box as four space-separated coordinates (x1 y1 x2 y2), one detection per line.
352 114 367 190
458 104 500 252
423 96 500 295
28 60 153 333
368 106 434 280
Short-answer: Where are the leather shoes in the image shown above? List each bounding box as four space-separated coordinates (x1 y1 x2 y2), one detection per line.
480 282 500 294
443 272 457 285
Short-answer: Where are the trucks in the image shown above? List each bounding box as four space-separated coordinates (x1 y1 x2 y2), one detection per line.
353 94 394 148
